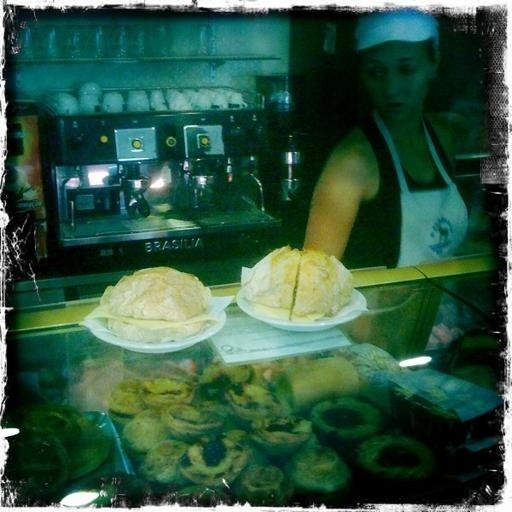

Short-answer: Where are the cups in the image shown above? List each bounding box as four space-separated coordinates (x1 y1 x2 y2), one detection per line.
48 80 248 115
479 185 507 259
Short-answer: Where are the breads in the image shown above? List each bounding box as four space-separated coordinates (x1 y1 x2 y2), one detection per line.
240 246 354 323
85 266 221 344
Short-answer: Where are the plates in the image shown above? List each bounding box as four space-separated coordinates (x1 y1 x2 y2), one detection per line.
237 285 368 334
85 306 228 356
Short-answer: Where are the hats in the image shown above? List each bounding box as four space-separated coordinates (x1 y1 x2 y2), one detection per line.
351 8 442 56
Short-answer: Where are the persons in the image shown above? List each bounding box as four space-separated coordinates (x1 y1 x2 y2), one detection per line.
301 8 491 361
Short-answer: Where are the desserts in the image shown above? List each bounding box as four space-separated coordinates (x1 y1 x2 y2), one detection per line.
107 357 434 506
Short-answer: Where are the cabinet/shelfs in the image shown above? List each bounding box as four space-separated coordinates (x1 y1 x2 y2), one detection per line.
9 251 499 506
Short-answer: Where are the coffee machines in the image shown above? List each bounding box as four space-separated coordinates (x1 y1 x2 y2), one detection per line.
255 73 311 211
41 116 284 269
